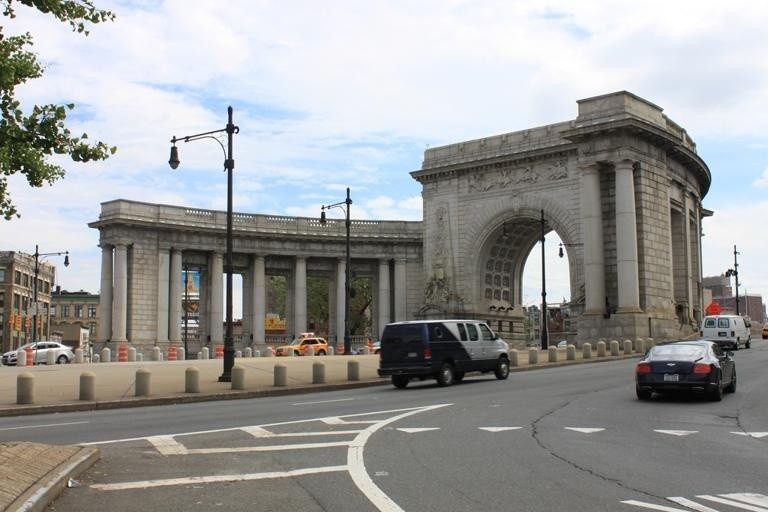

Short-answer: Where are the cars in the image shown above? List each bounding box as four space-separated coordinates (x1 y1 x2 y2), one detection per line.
275 333 328 356
636 340 736 401
357 338 380 354
2 341 74 365
762 322 768 338
558 340 567 348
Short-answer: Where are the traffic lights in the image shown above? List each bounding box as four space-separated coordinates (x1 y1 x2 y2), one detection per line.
725 269 736 277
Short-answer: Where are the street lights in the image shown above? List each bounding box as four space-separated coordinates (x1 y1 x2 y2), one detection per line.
168 106 239 382
503 209 548 349
32 244 69 341
320 187 353 355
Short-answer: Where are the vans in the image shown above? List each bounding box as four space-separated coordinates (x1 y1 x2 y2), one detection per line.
699 314 751 350
377 319 511 388
